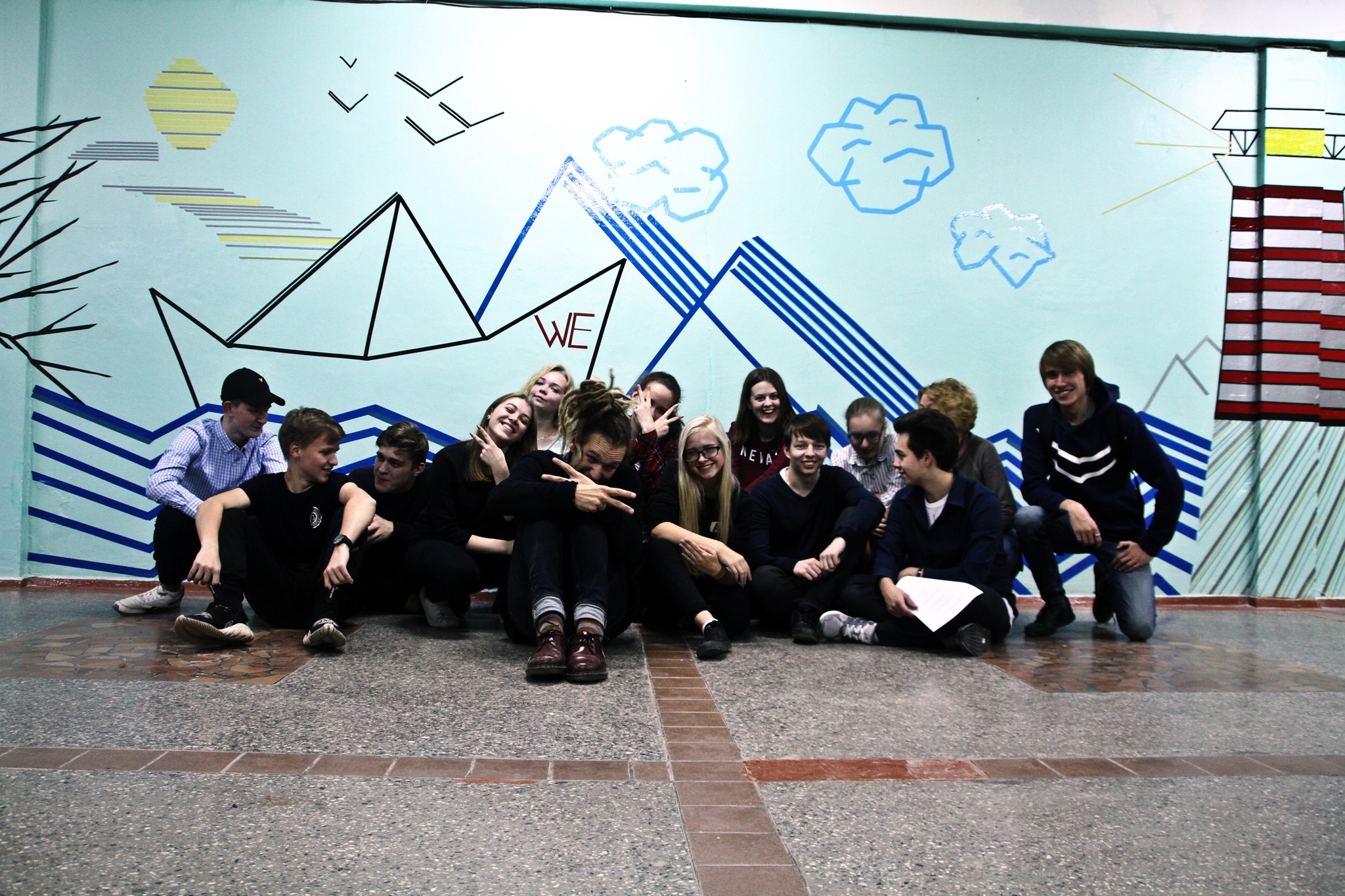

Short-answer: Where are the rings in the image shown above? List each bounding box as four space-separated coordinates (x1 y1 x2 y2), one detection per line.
1093 533 1101 536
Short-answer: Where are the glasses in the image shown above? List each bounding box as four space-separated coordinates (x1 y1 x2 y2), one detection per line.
683 443 722 462
847 424 885 445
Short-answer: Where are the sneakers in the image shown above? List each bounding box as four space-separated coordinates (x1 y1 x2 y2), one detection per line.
953 620 992 656
419 587 454 627
818 611 878 645
695 620 732 658
112 582 185 615
174 603 254 643
303 618 346 647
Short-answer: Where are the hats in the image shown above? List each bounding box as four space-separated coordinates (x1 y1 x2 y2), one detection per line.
221 368 285 408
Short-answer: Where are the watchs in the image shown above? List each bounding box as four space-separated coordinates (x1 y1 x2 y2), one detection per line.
333 534 352 549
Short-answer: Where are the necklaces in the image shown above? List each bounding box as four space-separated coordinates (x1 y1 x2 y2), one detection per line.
536 427 555 440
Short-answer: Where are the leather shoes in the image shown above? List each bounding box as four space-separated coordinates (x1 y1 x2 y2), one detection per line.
565 630 607 681
525 621 566 680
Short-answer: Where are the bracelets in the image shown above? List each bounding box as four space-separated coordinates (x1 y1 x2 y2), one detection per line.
916 568 922 576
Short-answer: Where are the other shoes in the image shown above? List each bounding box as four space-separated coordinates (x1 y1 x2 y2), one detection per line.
1093 561 1114 624
791 606 821 644
1023 602 1076 636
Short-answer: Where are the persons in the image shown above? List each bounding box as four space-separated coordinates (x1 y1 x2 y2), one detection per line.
349 364 1018 682
1014 340 1185 643
112 367 285 623
172 408 377 650
